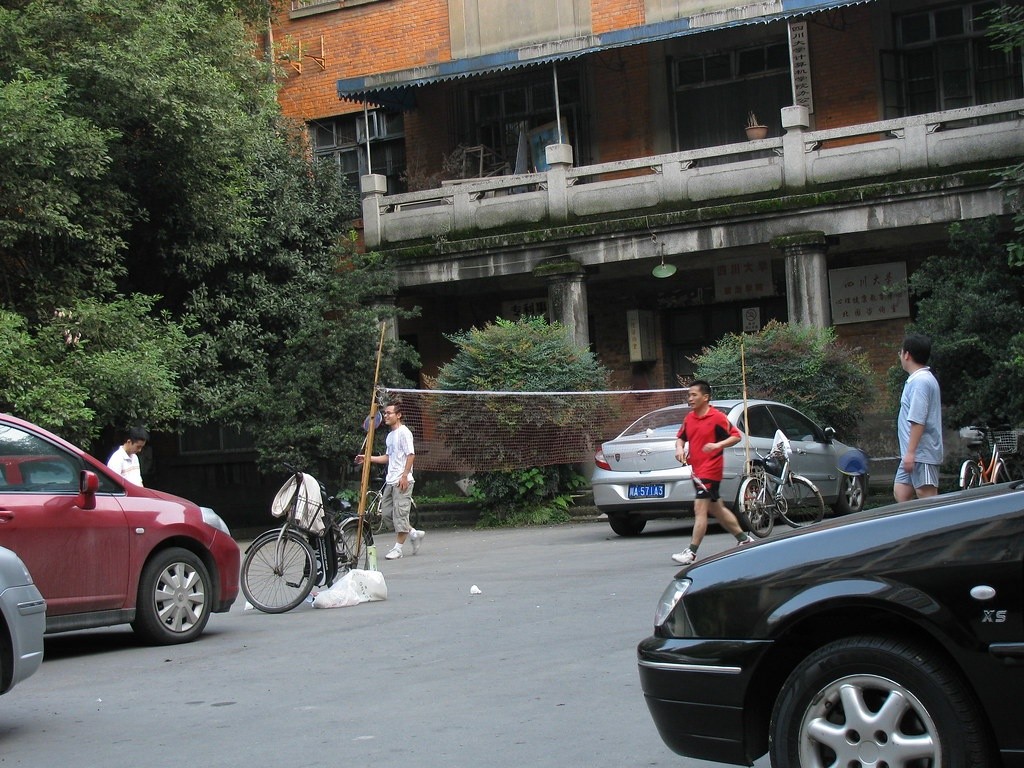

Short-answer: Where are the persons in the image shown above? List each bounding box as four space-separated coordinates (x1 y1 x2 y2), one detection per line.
353 402 425 560
671 380 755 565
107 427 150 489
892 335 944 504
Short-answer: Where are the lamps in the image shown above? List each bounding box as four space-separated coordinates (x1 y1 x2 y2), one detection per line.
652 241 676 278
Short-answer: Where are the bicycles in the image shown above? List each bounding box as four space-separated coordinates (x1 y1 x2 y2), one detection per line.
363 475 420 535
739 443 827 539
241 462 374 615
956 423 1020 490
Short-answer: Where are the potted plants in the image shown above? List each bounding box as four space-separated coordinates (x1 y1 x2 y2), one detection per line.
745 110 767 140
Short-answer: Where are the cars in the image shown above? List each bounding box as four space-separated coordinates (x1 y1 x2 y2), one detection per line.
0 548 48 696
591 397 872 537
636 472 1024 768
0 412 241 645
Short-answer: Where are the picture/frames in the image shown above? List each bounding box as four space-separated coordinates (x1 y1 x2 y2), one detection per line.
527 117 570 173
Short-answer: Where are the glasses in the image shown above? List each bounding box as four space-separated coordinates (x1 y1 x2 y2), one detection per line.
898 351 901 356
382 411 395 416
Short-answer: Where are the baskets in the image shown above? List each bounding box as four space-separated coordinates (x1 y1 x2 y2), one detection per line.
291 497 338 537
987 431 1018 454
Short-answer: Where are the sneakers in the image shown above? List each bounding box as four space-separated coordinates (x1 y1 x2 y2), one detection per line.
385 546 403 560
736 535 754 546
671 548 697 564
411 530 425 555
